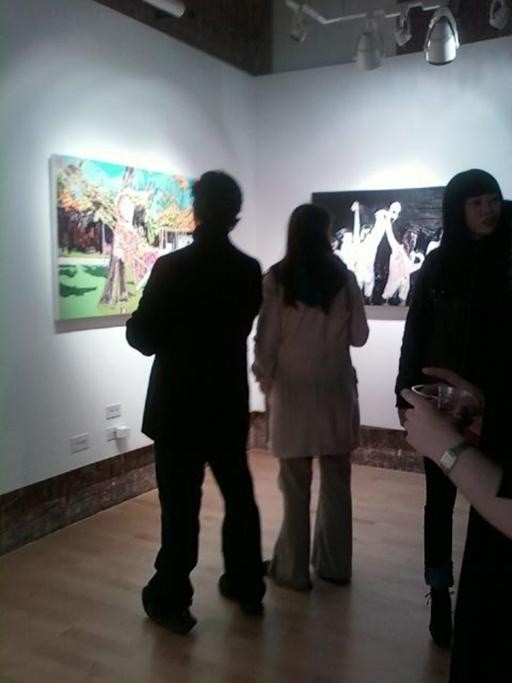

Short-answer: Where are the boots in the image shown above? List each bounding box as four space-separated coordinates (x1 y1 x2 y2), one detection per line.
429 587 452 649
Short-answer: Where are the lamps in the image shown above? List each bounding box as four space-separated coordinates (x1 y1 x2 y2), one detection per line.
486 0 511 30
351 4 462 71
287 0 309 43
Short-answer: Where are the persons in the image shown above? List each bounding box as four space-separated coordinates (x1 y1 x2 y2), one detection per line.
124 168 268 637
392 166 510 649
398 365 511 683
251 201 369 594
329 199 443 305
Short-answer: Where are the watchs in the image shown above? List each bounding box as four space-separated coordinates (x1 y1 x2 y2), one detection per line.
439 439 474 477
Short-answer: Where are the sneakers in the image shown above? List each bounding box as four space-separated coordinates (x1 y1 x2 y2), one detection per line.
263 560 312 591
314 563 352 587
141 586 197 634
218 571 266 604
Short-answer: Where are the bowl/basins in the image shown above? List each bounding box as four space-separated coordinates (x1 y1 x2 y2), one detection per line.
411 384 485 421
